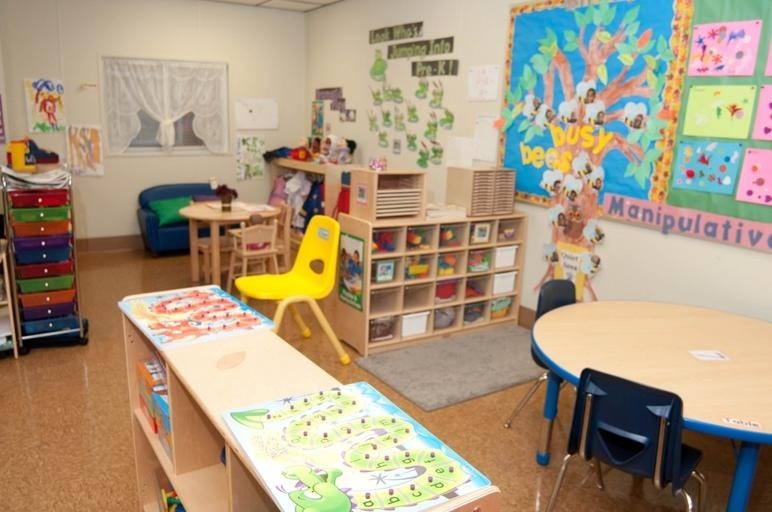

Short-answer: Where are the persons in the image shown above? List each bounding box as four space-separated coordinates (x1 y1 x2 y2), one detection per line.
544 161 604 271
349 249 360 274
339 247 351 277
529 88 643 130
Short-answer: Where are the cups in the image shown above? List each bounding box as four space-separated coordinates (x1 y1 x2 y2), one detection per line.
222 195 231 211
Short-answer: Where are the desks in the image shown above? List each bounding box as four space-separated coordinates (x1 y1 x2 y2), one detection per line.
530 298 772 511
179 201 282 286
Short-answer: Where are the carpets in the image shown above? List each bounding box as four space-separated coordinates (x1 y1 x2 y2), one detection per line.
352 322 549 412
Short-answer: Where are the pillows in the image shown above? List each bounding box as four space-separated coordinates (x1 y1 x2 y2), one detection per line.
149 194 221 228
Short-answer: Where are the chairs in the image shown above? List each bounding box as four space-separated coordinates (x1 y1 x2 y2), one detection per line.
505 278 579 427
551 367 706 510
233 214 351 365
188 199 293 295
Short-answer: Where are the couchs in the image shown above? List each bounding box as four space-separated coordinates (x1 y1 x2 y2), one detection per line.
137 183 240 258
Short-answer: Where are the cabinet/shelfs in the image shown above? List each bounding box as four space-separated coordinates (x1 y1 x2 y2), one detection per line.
320 211 529 357
270 156 356 251
2 176 88 357
114 284 517 511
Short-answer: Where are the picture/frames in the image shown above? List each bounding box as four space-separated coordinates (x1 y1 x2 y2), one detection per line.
66 123 104 176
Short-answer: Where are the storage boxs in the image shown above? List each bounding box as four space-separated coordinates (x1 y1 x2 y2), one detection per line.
368 218 522 342
